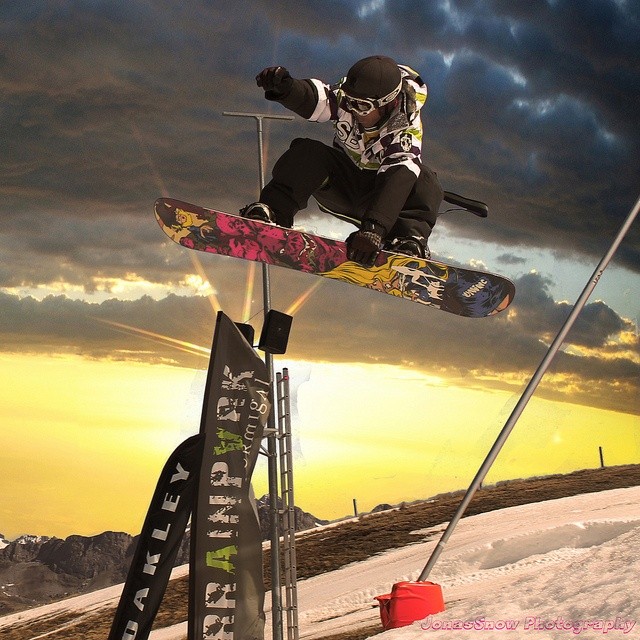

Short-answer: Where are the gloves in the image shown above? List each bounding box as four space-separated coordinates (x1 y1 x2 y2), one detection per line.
255 67 295 102
344 222 383 266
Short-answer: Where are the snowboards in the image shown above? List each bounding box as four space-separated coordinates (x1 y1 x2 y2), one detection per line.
153 197 516 319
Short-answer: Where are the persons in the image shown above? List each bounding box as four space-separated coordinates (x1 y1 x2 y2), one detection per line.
241 51 442 266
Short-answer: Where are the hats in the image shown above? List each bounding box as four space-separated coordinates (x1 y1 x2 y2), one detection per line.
343 54 401 98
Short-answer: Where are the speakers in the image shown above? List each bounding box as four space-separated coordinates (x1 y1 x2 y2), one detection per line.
258 308 293 354
233 322 255 349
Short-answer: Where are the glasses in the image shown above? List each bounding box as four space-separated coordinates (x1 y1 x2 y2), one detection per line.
337 77 403 117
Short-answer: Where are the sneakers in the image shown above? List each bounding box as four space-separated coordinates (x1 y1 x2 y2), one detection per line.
392 237 427 258
239 200 275 223
